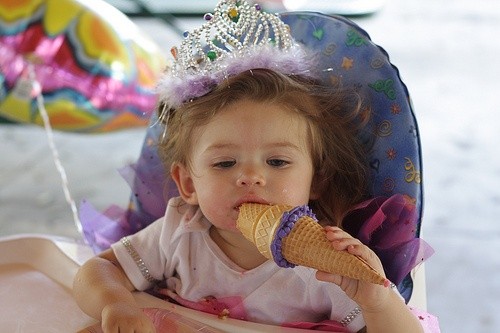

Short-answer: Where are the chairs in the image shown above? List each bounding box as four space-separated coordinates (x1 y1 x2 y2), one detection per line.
122 10 428 333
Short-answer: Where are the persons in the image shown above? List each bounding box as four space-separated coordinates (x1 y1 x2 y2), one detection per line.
72 0 427 333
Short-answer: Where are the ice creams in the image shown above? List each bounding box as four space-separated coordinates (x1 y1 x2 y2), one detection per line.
237 203 394 288
